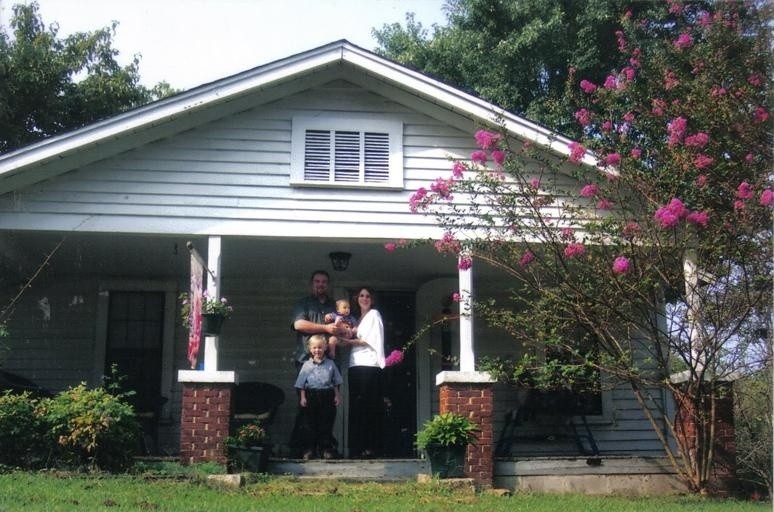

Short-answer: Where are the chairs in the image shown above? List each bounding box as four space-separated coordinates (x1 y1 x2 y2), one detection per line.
128 385 286 454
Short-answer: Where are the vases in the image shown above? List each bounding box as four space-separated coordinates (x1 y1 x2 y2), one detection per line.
200 314 225 337
233 447 266 474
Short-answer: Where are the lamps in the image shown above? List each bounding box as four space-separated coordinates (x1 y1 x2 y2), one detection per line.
329 250 351 271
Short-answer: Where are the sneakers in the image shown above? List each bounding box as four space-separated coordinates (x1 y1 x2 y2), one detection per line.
298 448 343 460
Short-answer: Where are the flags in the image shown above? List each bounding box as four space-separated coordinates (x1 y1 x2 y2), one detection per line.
186 248 206 368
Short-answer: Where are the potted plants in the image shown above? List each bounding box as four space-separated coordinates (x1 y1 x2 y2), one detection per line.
415 412 483 477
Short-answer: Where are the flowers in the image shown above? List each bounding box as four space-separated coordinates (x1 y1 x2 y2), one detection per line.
201 291 234 326
405 8 770 279
234 425 265 446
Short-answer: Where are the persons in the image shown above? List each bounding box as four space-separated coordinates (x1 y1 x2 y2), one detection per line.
288 270 353 458
332 285 386 459
293 333 344 460
322 298 359 361
